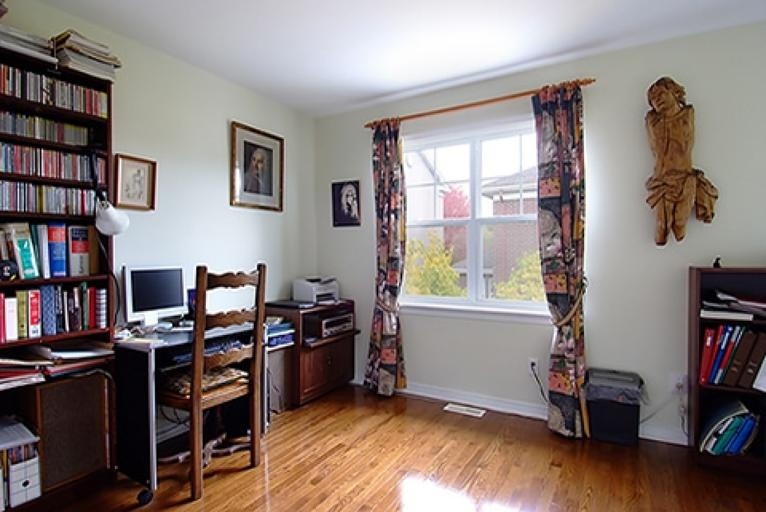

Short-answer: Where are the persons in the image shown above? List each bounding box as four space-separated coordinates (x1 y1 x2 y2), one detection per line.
640 75 698 246
243 147 272 196
336 184 359 224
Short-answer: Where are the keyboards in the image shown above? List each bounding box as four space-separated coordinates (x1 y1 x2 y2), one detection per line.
171 338 241 363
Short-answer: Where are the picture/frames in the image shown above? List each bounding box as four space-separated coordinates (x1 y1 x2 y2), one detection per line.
229 120 284 211
330 180 361 227
112 152 156 211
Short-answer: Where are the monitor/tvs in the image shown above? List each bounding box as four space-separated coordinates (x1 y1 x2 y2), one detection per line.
124 266 189 333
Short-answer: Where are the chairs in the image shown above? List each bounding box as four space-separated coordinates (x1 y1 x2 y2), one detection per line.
156 259 264 498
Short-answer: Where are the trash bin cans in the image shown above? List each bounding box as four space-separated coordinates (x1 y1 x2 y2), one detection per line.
586 368 640 446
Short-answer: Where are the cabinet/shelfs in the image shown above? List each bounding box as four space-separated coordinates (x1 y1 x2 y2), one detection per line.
0 48 118 512
686 265 766 485
265 301 361 408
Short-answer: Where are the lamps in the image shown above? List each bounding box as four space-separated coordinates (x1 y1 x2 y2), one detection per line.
92 186 128 337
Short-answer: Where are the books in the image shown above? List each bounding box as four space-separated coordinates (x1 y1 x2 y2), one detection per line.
126 337 161 343
0 415 40 512
1 222 106 343
699 283 766 459
1 344 115 391
1 64 108 215
0 24 121 83
119 341 169 349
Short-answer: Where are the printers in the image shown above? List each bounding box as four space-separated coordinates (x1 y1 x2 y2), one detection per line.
294 277 344 306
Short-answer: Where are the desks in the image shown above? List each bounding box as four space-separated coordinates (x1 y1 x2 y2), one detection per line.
115 321 270 502
